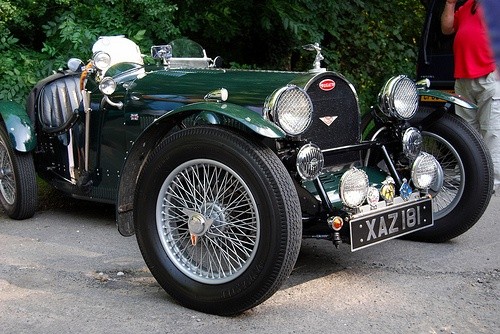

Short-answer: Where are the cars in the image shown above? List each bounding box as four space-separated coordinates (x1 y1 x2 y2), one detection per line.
0 34 496 318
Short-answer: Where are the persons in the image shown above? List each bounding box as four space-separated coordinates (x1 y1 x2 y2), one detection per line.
439 0 500 197
302 42 324 69
